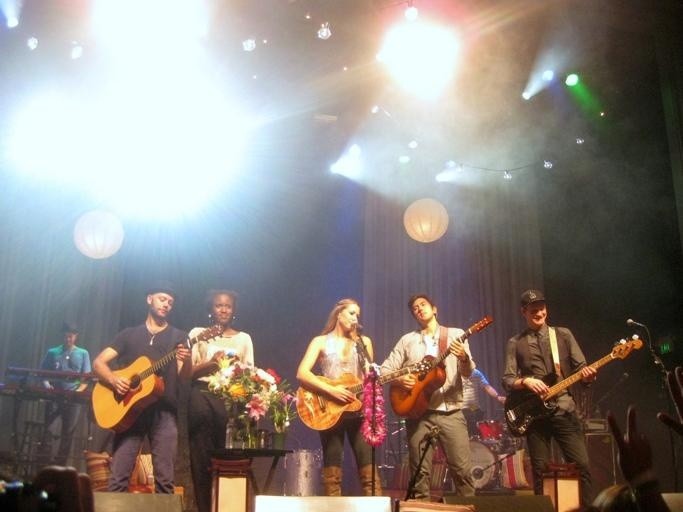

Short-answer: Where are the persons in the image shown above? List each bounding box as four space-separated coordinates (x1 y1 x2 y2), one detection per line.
93 287 191 493
187 290 254 512
296 298 383 496
38 319 91 467
380 294 476 502
592 405 671 512
461 368 507 438
501 290 597 504
32 464 95 512
657 367 682 437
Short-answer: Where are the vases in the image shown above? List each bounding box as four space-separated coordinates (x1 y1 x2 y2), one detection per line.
224 418 286 450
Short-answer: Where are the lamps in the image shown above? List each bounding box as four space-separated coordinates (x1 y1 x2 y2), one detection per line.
402 194 449 244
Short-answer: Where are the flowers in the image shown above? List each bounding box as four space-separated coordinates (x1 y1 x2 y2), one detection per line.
206 354 298 433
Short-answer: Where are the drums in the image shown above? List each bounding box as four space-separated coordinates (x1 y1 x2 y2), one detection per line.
284 449 322 496
477 420 505 452
468 437 501 490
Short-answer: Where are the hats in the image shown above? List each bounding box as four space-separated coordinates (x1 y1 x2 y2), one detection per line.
60 321 79 334
146 279 177 299
520 289 545 309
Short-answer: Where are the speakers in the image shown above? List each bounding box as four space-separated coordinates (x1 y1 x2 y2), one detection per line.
584 434 617 505
252 494 400 512
93 492 183 512
473 495 554 512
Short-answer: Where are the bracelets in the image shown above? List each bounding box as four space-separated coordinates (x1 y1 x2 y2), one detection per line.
630 469 659 496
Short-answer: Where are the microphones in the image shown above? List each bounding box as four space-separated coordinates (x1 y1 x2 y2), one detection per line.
626 318 646 328
351 322 363 331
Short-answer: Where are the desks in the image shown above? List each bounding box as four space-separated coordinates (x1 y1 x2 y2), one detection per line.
208 449 293 494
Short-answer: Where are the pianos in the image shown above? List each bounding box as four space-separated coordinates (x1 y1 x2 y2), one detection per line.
0 367 91 403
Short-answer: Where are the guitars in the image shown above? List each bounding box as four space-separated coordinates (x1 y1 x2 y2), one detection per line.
92 324 224 431
504 335 642 437
296 361 432 430
390 315 494 418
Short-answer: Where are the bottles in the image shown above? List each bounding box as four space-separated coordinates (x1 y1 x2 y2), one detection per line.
224 421 268 449
